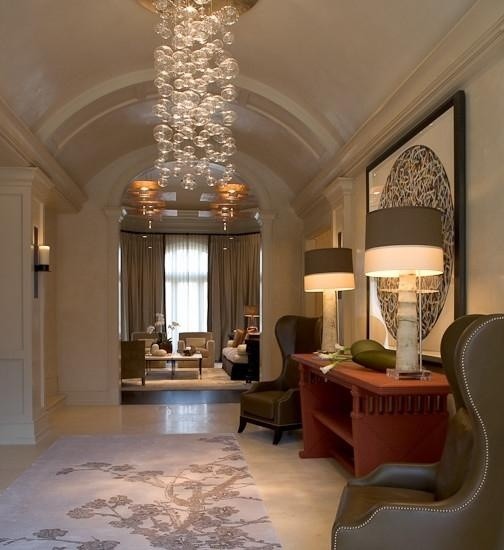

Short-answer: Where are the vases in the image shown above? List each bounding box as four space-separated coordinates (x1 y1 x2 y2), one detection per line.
161 341 172 352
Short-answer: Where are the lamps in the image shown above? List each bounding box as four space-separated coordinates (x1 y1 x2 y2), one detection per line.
363 206 446 382
36 245 51 271
244 305 260 327
152 0 239 192
303 247 357 357
132 180 163 227
212 177 244 230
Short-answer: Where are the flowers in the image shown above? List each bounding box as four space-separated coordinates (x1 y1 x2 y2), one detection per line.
147 313 178 341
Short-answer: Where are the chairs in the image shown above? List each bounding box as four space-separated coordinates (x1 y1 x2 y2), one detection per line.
121 340 148 384
237 314 322 445
331 313 503 550
178 332 215 368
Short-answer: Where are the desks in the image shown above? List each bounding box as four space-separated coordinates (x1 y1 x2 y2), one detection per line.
290 350 451 480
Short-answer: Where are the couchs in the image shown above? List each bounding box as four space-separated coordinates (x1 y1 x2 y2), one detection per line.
222 334 259 383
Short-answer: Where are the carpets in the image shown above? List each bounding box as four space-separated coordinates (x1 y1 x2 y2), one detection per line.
0 433 281 550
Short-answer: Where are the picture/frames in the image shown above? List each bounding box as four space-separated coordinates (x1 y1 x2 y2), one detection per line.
364 91 465 368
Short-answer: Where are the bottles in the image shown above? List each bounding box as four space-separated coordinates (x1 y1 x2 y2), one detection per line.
152 344 159 356
